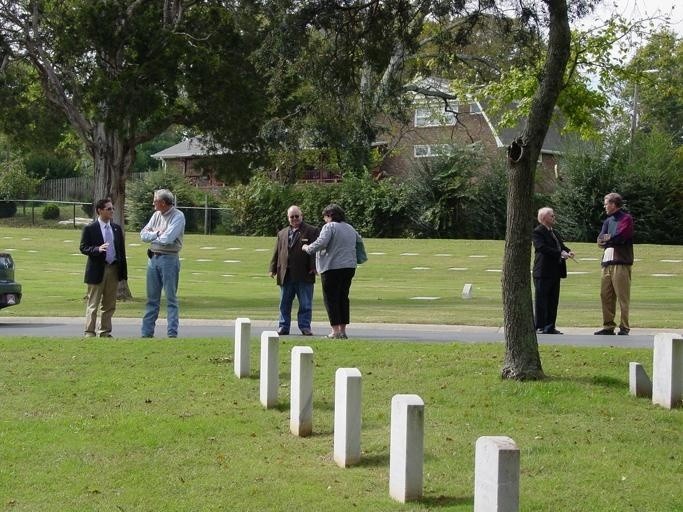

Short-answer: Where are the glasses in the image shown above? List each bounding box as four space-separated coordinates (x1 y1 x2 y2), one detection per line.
103 207 115 211
290 215 299 218
551 214 555 217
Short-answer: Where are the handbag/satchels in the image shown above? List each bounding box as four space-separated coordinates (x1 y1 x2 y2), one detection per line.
147 248 153 258
356 241 368 265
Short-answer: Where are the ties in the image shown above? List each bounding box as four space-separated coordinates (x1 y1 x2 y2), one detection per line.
104 225 114 264
550 230 553 235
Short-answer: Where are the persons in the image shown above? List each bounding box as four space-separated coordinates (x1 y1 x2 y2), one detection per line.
139 189 185 337
301 204 367 338
267 206 320 335
593 192 634 335
532 208 575 334
77 198 127 338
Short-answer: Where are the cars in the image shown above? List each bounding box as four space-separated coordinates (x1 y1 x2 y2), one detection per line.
0 253 22 309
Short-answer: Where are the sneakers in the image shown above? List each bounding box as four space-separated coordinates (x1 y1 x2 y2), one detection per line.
323 334 348 339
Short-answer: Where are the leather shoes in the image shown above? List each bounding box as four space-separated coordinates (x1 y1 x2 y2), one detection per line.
277 327 290 335
594 328 629 335
536 327 560 333
302 330 312 335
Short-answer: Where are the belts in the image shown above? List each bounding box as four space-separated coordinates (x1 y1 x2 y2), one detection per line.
105 261 116 265
